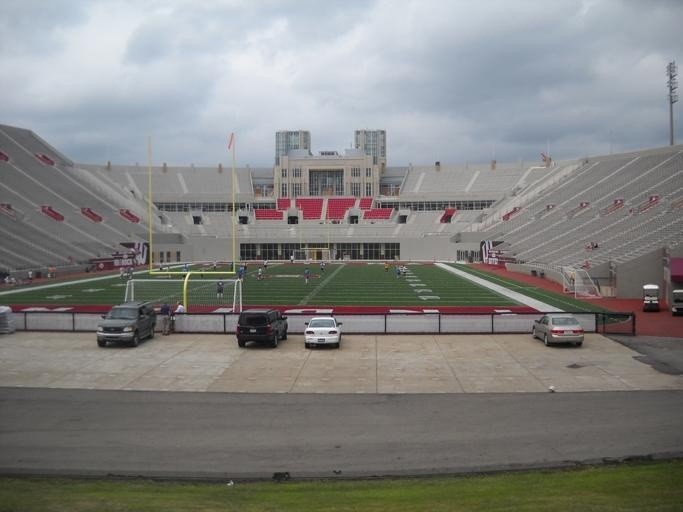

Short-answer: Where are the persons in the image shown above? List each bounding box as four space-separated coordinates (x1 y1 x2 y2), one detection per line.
28 270 33 282
213 259 217 271
182 264 189 279
320 262 325 271
290 255 294 263
383 261 408 279
303 267 310 285
120 265 134 279
216 278 224 300
160 300 186 338
257 259 268 281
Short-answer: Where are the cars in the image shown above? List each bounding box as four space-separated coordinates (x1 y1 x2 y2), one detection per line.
533 314 584 346
304 317 342 348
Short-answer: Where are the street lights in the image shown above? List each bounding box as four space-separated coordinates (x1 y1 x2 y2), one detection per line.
666 63 678 145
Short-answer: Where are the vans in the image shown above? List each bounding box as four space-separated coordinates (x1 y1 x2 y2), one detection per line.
236 309 287 348
97 302 156 347
